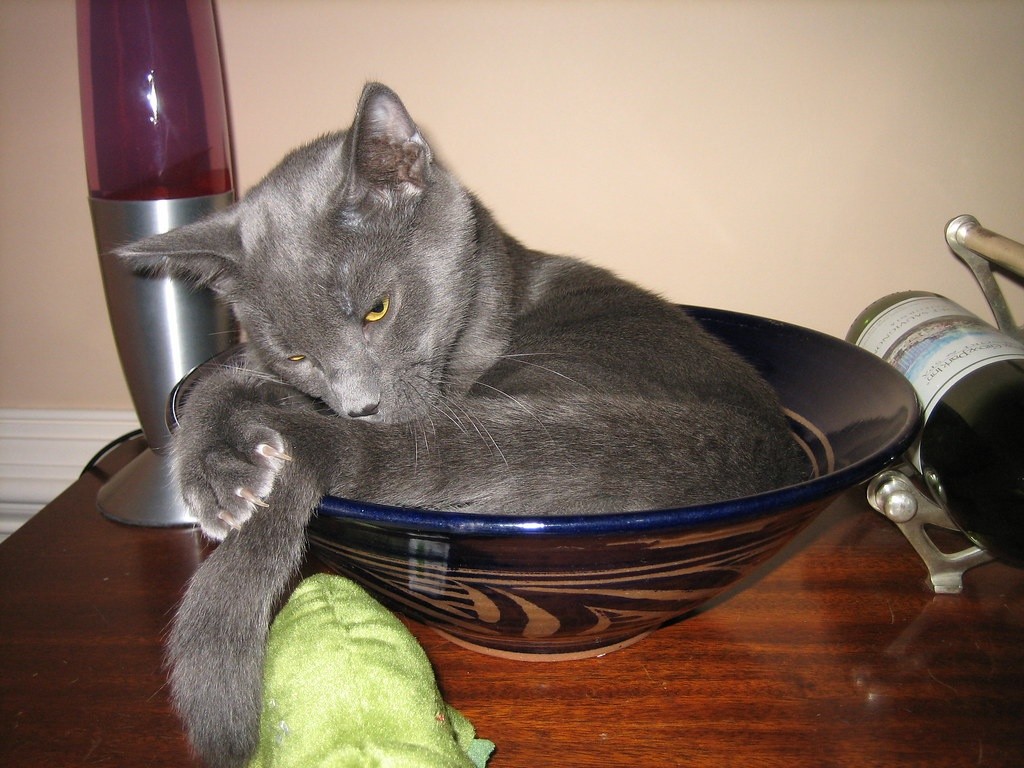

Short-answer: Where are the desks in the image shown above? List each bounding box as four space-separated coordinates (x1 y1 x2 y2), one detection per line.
0 432 1024 768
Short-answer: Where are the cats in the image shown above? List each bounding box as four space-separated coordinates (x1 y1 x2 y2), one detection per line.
107 81 797 765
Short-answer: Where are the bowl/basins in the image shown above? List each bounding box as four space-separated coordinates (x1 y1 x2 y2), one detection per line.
166 303 924 664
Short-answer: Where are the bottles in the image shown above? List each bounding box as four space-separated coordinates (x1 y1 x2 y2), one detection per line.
845 291 1024 570
